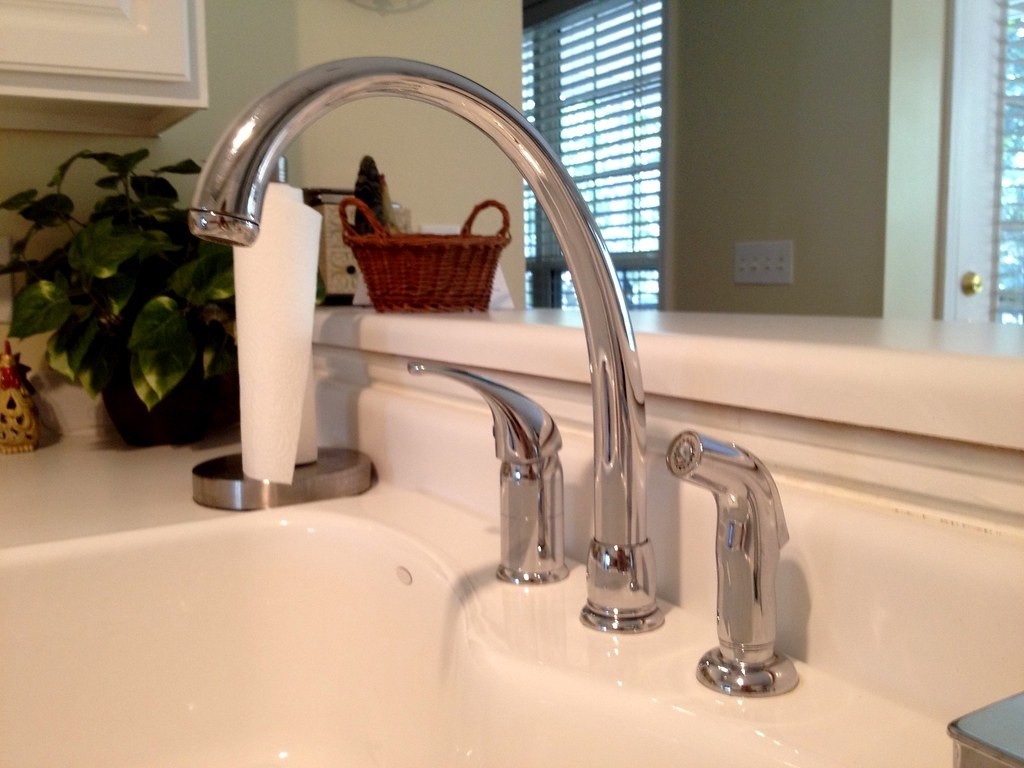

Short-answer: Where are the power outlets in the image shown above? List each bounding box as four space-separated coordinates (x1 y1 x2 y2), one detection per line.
733 239 795 285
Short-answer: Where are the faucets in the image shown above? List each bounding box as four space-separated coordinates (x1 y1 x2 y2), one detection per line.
186 54 801 700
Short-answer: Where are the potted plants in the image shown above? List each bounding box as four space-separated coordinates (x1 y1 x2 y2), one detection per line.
0 149 241 450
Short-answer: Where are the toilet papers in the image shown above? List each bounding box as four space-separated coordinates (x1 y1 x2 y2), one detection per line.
230 179 323 490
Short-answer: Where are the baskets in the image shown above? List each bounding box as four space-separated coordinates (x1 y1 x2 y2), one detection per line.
338 197 510 312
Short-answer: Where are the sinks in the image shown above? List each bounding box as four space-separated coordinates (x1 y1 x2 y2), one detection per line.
0 508 955 767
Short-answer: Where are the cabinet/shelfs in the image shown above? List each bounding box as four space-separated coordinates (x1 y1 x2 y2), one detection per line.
0 0 211 139
